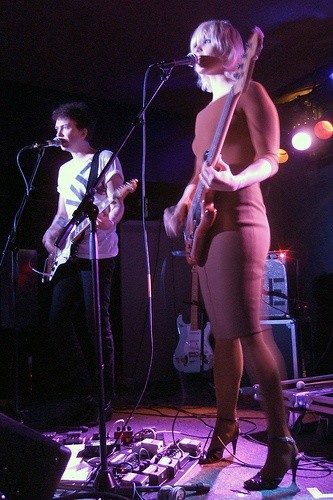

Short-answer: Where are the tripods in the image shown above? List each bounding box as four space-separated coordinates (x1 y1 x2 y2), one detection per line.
54 67 212 500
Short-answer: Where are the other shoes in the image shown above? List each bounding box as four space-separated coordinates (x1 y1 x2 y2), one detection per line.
86 399 113 426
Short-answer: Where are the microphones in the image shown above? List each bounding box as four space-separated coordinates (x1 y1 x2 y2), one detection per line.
31 137 62 147
158 53 198 69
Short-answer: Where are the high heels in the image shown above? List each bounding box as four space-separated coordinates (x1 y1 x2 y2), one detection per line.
197 416 240 464
244 435 301 491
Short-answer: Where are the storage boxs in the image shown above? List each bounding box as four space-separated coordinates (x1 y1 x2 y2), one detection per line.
258 320 299 380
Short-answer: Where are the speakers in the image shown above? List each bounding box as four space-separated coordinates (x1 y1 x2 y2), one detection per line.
0 414 71 500
259 259 287 317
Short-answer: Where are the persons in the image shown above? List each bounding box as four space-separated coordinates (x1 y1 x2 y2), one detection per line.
42 101 126 429
164 22 298 493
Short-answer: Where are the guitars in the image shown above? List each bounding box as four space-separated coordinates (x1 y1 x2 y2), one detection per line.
172 267 216 373
43 180 138 281
183 26 265 267
